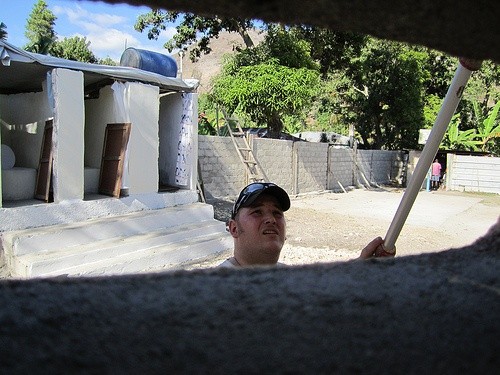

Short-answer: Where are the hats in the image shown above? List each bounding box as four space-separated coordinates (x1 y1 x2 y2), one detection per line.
231 182 291 221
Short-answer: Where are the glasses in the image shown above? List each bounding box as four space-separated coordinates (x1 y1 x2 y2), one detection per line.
235 184 277 213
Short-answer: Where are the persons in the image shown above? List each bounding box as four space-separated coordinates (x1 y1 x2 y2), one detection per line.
442 168 446 186
217 182 383 267
430 159 442 190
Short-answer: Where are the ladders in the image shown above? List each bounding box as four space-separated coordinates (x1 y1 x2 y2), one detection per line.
220 106 271 187
